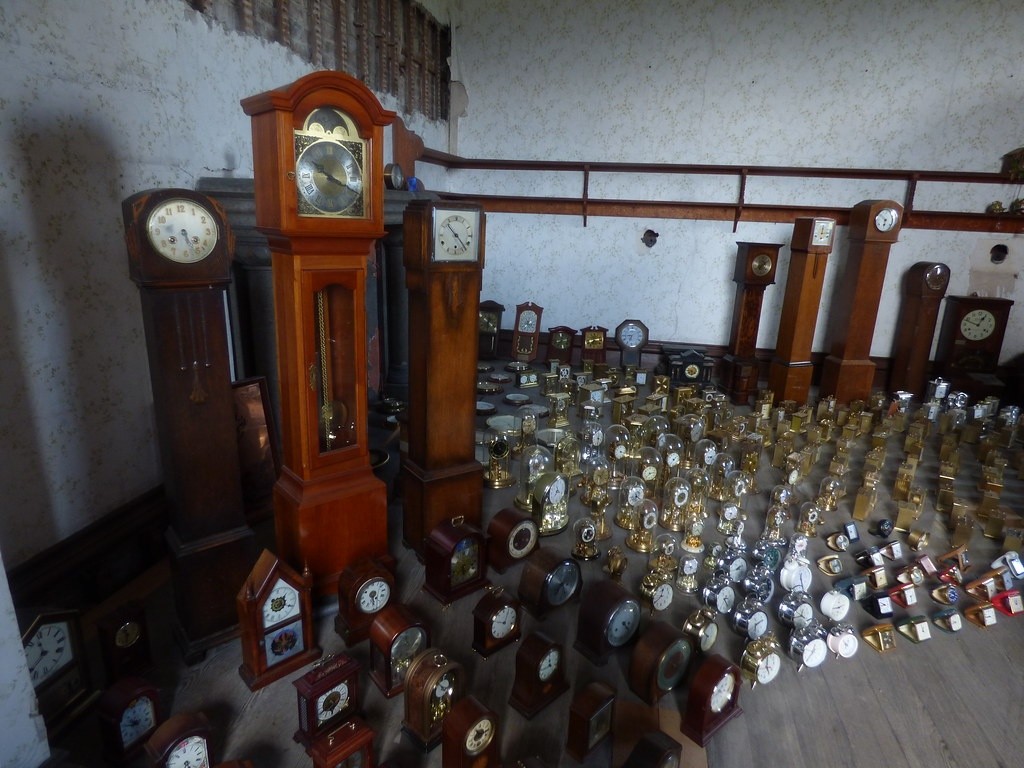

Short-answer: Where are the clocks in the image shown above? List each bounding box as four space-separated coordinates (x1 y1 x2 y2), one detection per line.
769 217 837 407
23 512 502 768
476 297 1024 768
124 187 259 554
402 200 487 546
885 262 951 402
820 200 903 402
240 72 398 596
718 243 785 404
